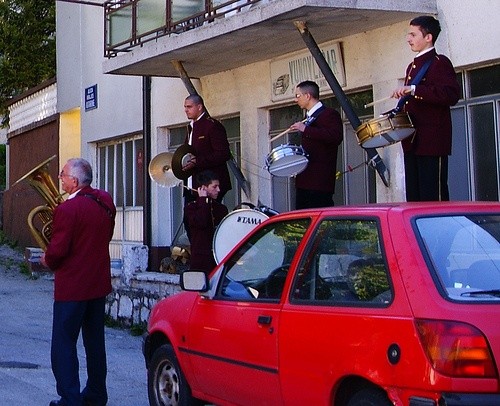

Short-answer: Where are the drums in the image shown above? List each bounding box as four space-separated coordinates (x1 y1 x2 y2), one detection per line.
262 142 311 179
211 202 281 268
354 112 415 149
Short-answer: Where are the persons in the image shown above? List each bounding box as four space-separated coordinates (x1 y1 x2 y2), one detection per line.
391 16 461 202
185 170 230 276
181 94 232 241
39 159 116 406
290 81 344 210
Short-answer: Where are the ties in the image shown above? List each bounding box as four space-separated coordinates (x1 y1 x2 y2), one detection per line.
307 116 309 123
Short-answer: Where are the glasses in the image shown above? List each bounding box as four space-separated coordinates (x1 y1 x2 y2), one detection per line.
60 170 77 179
295 93 307 98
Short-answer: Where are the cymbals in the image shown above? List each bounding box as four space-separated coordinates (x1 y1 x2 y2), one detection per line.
148 142 172 186
171 142 197 180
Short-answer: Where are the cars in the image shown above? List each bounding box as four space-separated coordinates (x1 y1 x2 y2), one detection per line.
141 201 499 402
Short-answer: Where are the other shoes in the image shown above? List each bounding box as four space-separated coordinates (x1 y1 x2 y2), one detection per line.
49 400 60 406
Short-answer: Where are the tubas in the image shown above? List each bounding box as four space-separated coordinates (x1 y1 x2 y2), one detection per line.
12 153 68 256
